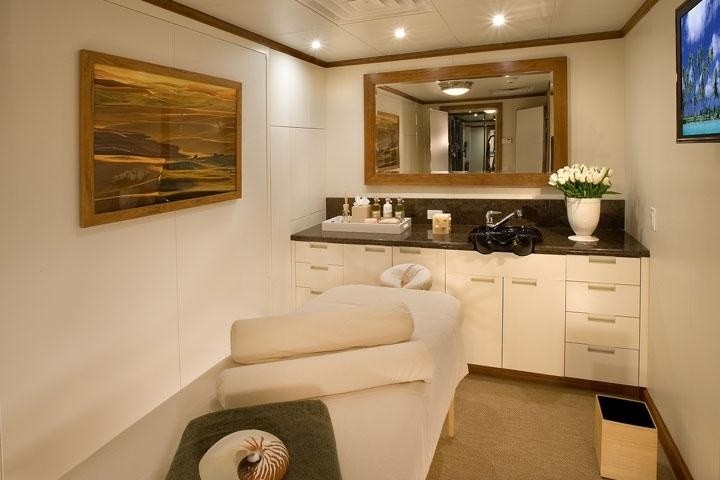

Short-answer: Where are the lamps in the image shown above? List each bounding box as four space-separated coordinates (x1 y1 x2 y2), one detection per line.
438 81 473 96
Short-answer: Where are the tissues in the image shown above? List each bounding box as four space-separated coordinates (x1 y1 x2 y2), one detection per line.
352 193 371 223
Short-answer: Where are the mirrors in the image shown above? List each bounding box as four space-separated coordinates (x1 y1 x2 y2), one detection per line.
364 55 568 188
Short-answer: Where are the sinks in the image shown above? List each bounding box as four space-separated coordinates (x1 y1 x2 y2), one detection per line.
468 226 544 256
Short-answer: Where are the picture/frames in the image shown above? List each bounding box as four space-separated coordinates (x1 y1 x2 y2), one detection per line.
375 111 400 172
675 1 719 143
79 48 243 227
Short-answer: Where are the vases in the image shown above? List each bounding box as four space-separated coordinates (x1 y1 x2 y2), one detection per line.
566 197 601 242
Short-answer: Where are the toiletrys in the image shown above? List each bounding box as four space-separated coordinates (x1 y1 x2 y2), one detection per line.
394 199 405 220
383 198 392 219
371 196 381 218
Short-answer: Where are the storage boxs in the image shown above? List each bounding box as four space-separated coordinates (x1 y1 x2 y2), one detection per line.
594 393 659 480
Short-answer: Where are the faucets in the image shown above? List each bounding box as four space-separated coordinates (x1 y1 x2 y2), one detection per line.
485 209 524 228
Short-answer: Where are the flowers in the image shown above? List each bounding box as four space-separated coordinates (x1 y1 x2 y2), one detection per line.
547 163 622 199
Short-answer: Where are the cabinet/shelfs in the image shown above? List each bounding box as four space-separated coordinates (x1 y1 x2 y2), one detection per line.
565 254 641 386
295 242 344 307
393 246 445 293
344 244 392 285
445 250 566 377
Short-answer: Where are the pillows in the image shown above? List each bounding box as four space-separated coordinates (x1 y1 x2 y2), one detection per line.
231 308 413 364
167 400 344 479
215 340 436 410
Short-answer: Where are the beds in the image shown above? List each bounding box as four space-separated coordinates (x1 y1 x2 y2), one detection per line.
59 286 470 480
